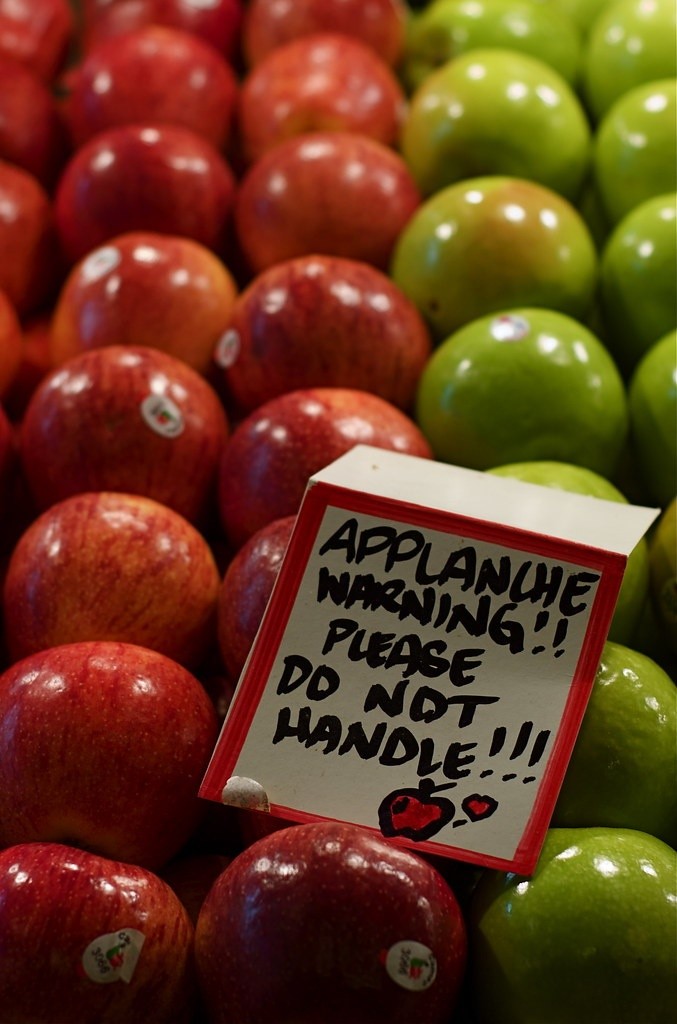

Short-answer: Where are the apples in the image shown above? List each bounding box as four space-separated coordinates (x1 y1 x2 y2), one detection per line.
0 0 677 1024
